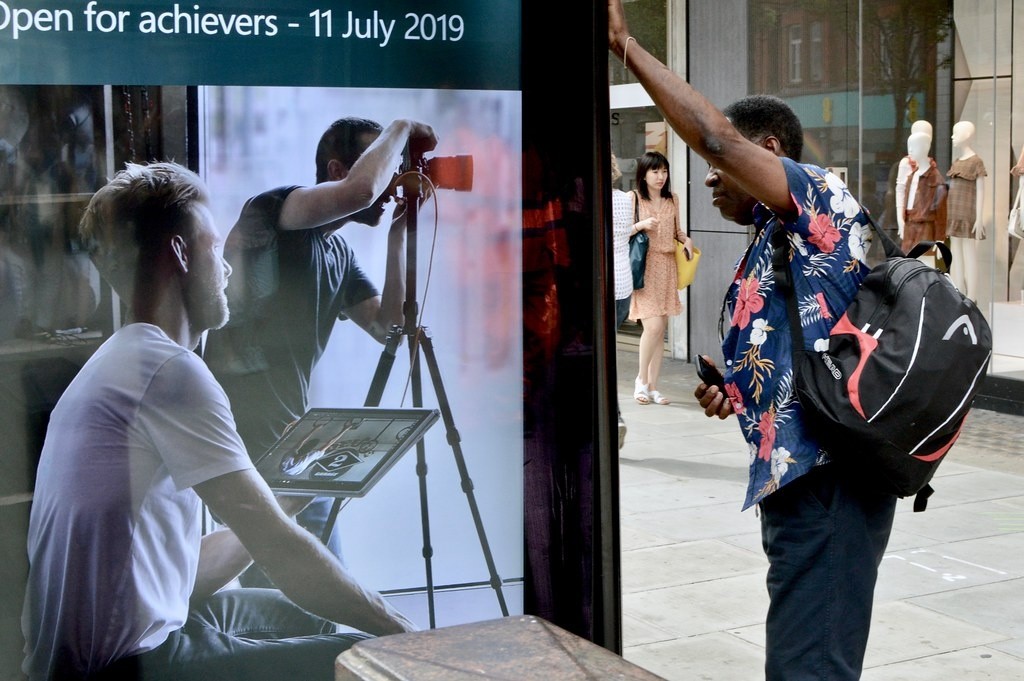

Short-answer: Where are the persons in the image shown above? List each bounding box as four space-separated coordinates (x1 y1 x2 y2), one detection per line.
22 157 424 681
611 152 633 332
626 150 699 405
280 423 353 476
945 120 988 305
608 1 899 681
204 117 440 584
895 119 947 271
1010 145 1024 232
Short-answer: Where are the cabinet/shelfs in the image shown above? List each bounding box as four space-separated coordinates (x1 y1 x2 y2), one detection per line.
0 83 119 680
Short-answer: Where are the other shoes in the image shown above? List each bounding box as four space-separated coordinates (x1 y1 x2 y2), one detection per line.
618 417 626 450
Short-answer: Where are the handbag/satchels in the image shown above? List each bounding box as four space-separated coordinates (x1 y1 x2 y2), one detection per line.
670 196 701 290
628 190 649 290
1008 208 1024 239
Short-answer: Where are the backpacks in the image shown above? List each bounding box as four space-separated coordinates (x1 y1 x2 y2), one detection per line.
771 196 993 513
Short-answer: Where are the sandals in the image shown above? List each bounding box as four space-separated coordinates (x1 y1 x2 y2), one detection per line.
634 376 650 404
649 391 670 405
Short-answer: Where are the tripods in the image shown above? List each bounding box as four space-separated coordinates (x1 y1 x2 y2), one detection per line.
319 192 510 634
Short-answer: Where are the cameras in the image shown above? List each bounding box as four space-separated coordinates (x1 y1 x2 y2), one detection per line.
400 139 474 195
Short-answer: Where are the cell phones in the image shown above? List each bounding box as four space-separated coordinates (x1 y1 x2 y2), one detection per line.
696 355 728 400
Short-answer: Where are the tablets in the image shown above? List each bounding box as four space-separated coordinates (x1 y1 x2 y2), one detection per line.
251 406 440 498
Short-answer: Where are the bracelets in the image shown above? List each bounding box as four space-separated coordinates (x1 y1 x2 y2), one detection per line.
634 224 640 232
623 37 636 69
